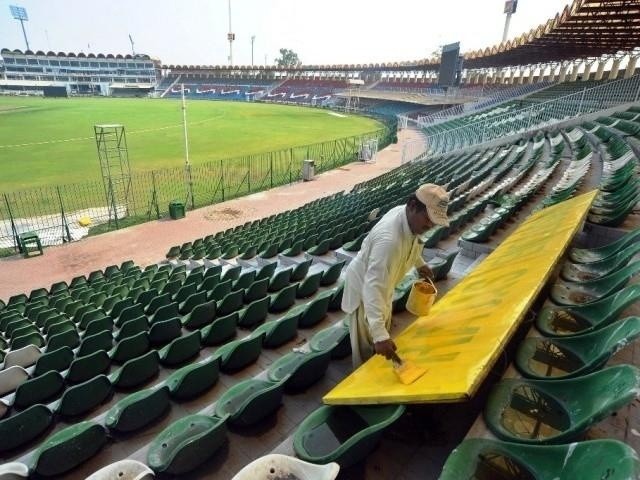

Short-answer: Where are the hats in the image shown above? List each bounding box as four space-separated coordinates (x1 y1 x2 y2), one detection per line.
415 183 450 228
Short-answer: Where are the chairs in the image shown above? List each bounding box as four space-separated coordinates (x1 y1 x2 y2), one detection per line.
222 242 236 253
296 226 304 233
292 258 313 282
252 219 260 228
217 238 229 244
92 277 106 281
78 309 108 328
169 264 186 277
549 260 640 306
239 245 259 259
106 331 152 364
0 299 6 310
60 349 113 385
172 282 197 302
216 288 245 317
263 75 640 225
30 288 48 297
199 312 239 345
321 260 347 284
256 240 271 255
354 222 370 236
191 247 206 260
370 217 382 231
112 285 129 298
292 233 307 245
239 241 252 253
51 281 70 292
0 365 30 398
260 217 268 225
281 221 289 227
225 228 233 237
0 404 54 451
152 271 169 280
85 459 156 480
0 462 28 480
107 297 134 317
88 291 108 304
80 316 113 339
44 314 70 331
271 222 280 227
149 316 182 348
181 241 193 254
29 305 49 319
74 282 90 288
277 227 289 234
40 329 80 351
254 236 265 245
342 314 349 326
182 300 217 332
215 331 266 373
98 293 122 309
362 212 371 220
271 226 277 231
418 226 446 249
147 302 178 327
78 287 96 299
195 243 207 254
354 216 364 226
0 313 23 331
256 261 278 280
29 346 75 378
120 274 136 285
0 309 20 318
297 270 324 298
147 414 232 474
37 308 59 325
136 288 158 304
144 292 171 315
0 344 43 372
75 330 113 357
267 231 277 240
71 287 88 298
191 265 205 273
272 235 285 244
307 237 333 256
163 280 182 293
68 275 87 287
259 229 268 236
269 282 300 311
559 242 640 283
215 231 224 240
207 279 232 301
245 277 270 304
268 267 293 292
6 369 66 411
254 228 264 232
55 296 75 308
303 235 320 250
317 281 345 310
128 287 145 303
268 342 337 395
233 235 241 241
278 238 293 253
267 214 277 224
109 270 120 276
244 221 253 229
317 224 330 232
437 438 639 479
105 350 161 387
471 218 503 236
180 248 194 260
263 224 272 231
73 303 97 322
0 338 7 349
47 375 110 419
106 385 170 433
168 272 186 283
220 265 242 283
288 220 299 225
207 244 220 253
330 224 343 237
294 405 409 467
319 220 327 224
299 221 307 226
120 261 134 270
197 274 221 292
287 293 334 325
1 397 10 418
113 303 144 328
247 234 258 242
260 243 282 258
194 238 203 250
0 331 3 338
105 265 120 277
285 231 295 238
342 232 371 251
231 455 338 480
236 296 272 327
140 270 153 280
234 224 244 233
281 238 306 257
290 224 298 232
101 281 118 295
342 219 354 231
150 278 167 292
156 328 201 366
53 288 70 295
221 245 239 258
183 272 203 286
9 293 28 303
166 245 181 258
309 222 317 228
206 247 222 259
88 270 104 281
145 264 158 271
232 269 257 290
251 311 304 346
484 364 639 443
515 316 640 378
132 278 150 290
7 302 24 310
306 228 317 237
396 274 417 287
317 228 332 243
12 324 39 336
412 250 458 281
12 333 44 350
110 274 123 282
342 227 358 245
126 270 141 278
24 301 43 316
5 318 32 338
220 239 233 247
49 293 68 305
179 290 207 314
329 233 346 250
461 220 497 243
214 373 294 424
535 284 640 336
203 235 214 245
329 221 335 226
112 315 149 342
91 281 105 288
29 420 108 476
0 349 7 363
64 300 85 313
159 264 172 273
47 320 77 337
243 231 253 240
310 327 351 360
126 266 140 277
237 238 245 244
165 354 222 400
392 286 411 311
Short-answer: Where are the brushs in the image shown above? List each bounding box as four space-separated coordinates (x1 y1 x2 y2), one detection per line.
390 351 428 383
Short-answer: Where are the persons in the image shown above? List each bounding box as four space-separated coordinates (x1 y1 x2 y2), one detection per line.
338 183 450 370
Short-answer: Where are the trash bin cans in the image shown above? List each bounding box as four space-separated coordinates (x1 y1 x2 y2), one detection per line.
304 159 317 184
